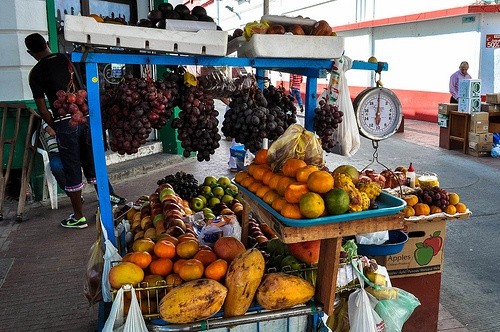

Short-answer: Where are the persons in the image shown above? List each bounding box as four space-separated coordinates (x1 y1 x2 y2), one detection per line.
39 106 88 205
449 61 472 104
24 33 127 229
289 73 304 112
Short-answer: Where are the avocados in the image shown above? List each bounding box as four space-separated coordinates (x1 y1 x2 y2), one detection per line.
137 3 222 29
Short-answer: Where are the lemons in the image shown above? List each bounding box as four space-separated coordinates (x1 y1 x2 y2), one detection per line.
368 57 377 62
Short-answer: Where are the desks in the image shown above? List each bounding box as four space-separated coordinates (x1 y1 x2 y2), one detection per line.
448 111 500 155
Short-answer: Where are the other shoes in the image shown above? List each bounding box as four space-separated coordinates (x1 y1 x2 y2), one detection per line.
110 192 127 204
81 196 85 204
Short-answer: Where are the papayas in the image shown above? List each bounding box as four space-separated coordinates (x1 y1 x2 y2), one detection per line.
157 279 228 324
222 247 265 328
254 273 315 310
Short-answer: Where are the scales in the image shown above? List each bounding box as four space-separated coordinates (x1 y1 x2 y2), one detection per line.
354 64 409 255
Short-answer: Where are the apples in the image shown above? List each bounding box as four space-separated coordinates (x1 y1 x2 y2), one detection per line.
265 20 333 36
109 176 301 312
235 150 333 220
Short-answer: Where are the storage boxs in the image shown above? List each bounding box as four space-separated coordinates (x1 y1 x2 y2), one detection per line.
438 114 448 128
458 98 481 114
457 78 481 99
470 112 489 134
237 34 344 59
468 132 493 151
486 93 500 104
165 16 216 33
371 220 446 277
481 102 499 113
439 126 448 149
63 13 228 56
438 103 458 115
466 147 488 157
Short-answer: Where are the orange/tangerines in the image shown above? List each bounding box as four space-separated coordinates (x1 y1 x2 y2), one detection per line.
299 192 325 218
324 188 350 215
403 192 466 217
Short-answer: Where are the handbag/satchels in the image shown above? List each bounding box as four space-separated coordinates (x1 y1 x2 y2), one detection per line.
82 207 123 307
326 259 421 332
316 56 360 157
266 124 326 173
102 284 148 332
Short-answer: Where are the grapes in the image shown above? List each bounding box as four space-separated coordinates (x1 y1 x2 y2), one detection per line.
408 186 450 210
52 89 89 127
157 170 205 201
100 73 343 162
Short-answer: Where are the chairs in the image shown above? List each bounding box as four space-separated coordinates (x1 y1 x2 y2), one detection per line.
32 130 85 210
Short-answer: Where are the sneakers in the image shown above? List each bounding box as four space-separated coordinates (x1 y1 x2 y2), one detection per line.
60 214 88 229
